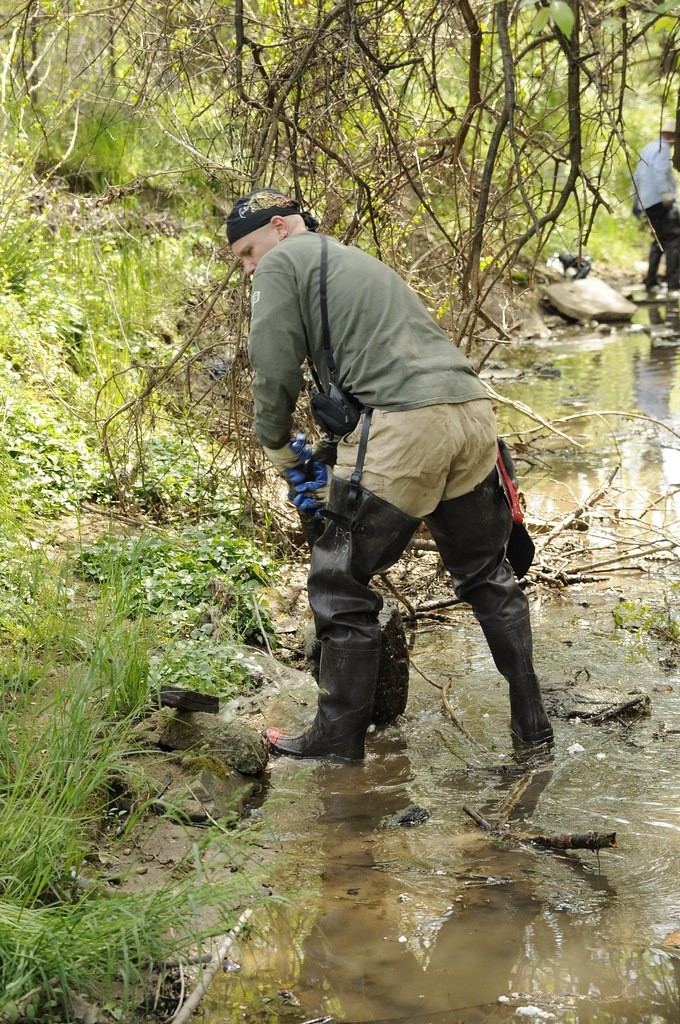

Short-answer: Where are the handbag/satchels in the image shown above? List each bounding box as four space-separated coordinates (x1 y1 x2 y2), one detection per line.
309 381 361 442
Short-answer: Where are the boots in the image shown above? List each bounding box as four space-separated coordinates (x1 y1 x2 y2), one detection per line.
645 211 680 291
265 477 423 762
421 438 555 745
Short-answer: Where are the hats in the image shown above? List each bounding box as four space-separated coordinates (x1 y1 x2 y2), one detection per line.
661 119 676 133
227 188 320 246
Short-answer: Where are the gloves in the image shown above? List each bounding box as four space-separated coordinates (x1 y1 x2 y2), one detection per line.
260 434 333 519
633 206 642 218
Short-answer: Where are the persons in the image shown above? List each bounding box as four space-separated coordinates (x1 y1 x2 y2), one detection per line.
226 188 555 763
631 118 680 300
